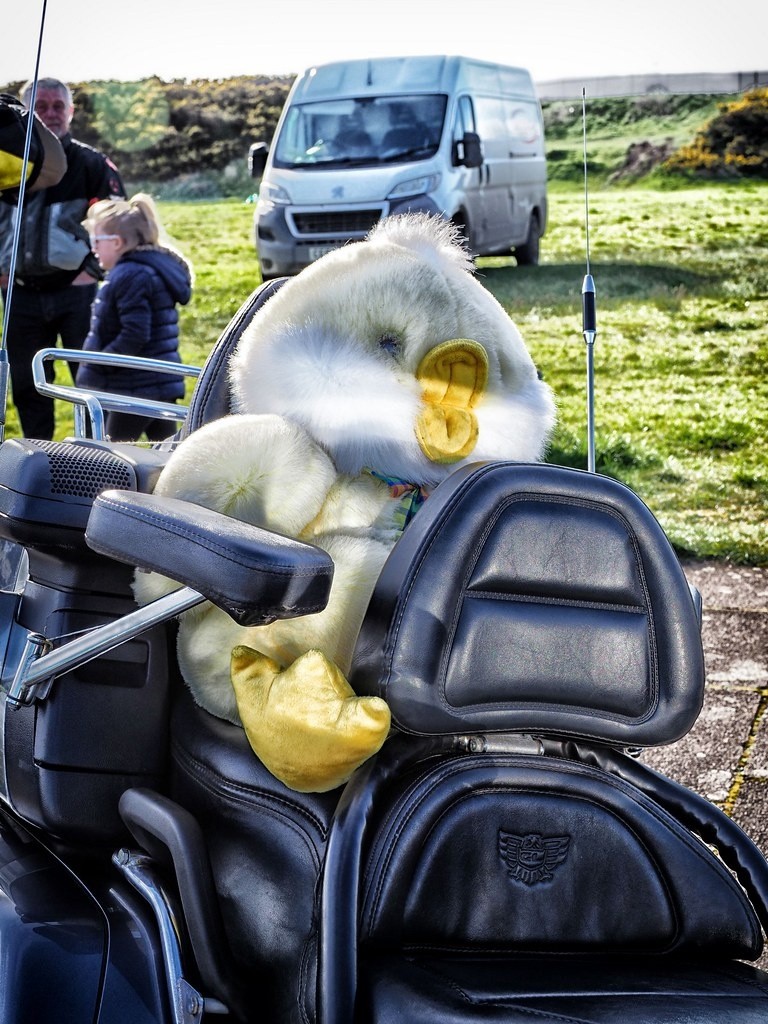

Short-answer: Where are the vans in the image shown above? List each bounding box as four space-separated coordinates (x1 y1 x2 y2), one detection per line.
244 54 550 288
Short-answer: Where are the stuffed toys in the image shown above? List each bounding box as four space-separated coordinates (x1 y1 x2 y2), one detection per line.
134 211 558 793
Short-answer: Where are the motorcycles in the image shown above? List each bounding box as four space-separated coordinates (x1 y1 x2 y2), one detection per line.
0 271 768 1024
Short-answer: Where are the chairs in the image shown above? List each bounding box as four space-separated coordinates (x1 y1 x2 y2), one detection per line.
300 460 768 1024
382 103 430 149
332 108 372 151
11 278 328 1024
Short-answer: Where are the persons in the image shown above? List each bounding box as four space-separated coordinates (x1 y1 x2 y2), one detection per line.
0 77 193 441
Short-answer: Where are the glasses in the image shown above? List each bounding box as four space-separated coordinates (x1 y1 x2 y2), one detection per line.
89 234 115 250
